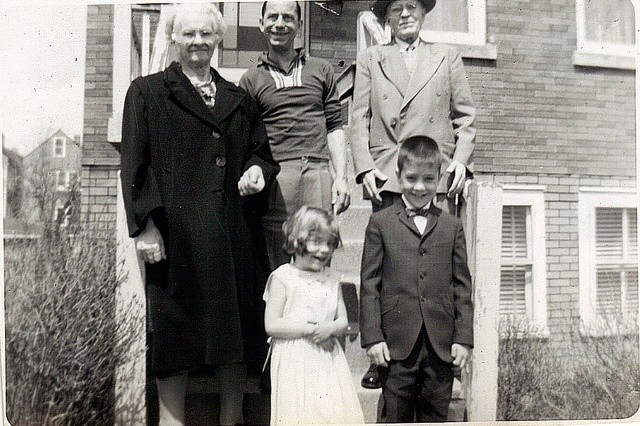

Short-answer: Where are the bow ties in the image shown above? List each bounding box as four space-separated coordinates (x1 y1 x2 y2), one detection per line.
407 207 431 217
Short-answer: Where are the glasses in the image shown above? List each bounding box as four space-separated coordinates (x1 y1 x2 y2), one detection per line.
386 2 424 13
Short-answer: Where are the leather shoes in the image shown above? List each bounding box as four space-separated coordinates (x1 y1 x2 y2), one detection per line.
362 365 382 388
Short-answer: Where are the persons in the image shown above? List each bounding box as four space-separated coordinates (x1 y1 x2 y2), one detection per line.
119 2 280 426
348 0 478 389
359 135 475 421
238 2 353 273
261 205 367 424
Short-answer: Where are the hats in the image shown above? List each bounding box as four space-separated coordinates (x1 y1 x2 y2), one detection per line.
371 0 436 18
